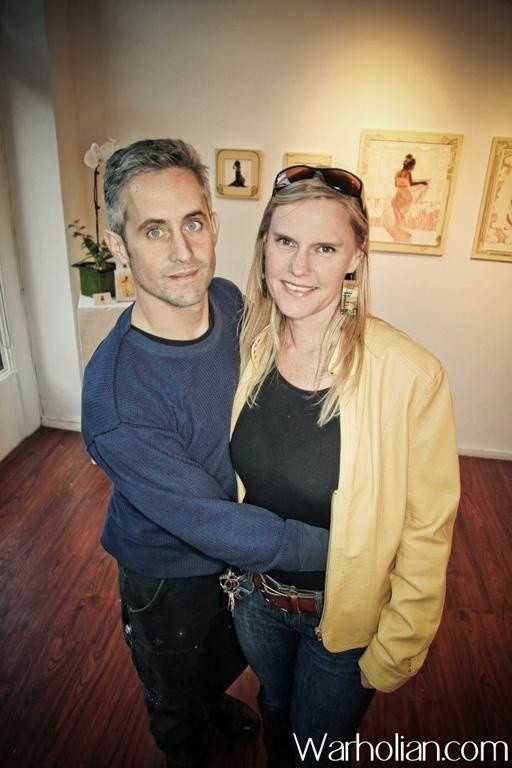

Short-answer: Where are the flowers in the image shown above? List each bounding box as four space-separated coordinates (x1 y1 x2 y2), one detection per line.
70 138 119 268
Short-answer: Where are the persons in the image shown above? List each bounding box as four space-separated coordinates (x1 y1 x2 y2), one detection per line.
389 154 428 228
221 163 462 767
80 139 329 768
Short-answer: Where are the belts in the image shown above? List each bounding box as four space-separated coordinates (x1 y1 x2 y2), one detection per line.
238 567 324 616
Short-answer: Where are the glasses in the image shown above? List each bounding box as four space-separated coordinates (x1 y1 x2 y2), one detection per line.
271 165 366 220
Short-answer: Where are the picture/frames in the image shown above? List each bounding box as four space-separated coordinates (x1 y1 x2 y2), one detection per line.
214 130 511 266
113 268 136 302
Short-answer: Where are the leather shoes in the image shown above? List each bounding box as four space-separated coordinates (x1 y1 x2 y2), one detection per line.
218 693 262 740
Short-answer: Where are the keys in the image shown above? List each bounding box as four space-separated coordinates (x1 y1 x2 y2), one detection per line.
220 566 255 619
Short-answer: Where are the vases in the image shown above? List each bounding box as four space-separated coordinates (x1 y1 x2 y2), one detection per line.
71 261 116 299
